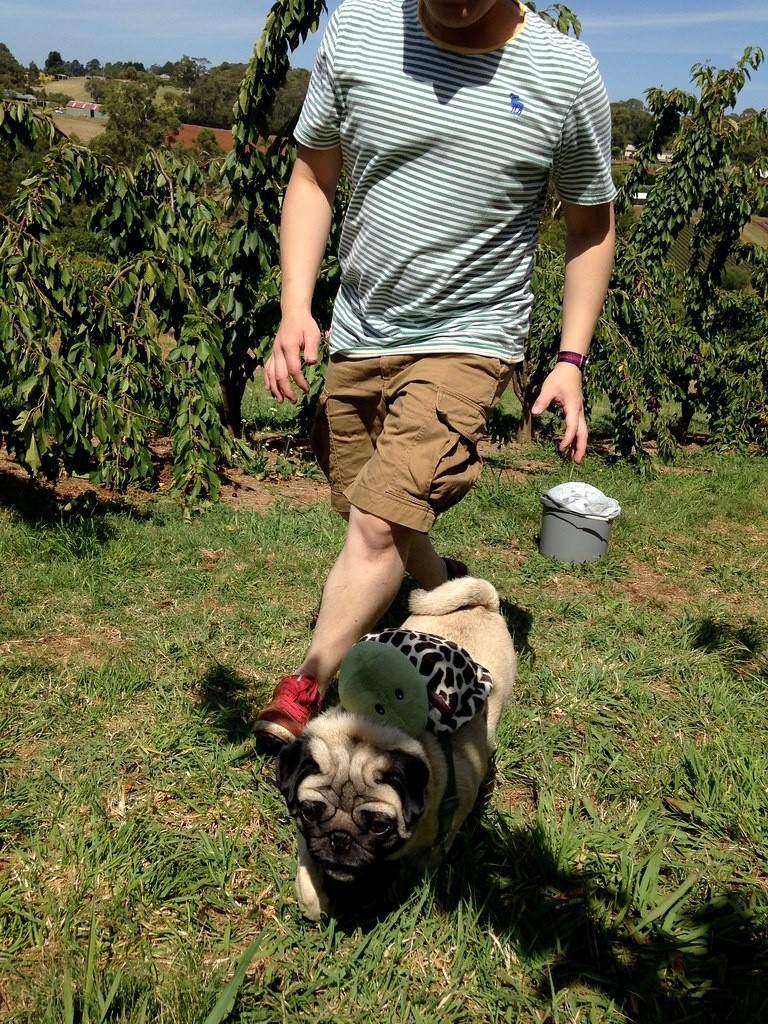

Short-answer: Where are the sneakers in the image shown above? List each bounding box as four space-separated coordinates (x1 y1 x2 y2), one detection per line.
390 557 470 620
251 674 322 754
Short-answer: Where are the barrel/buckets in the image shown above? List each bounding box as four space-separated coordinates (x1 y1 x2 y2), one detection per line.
540 492 611 569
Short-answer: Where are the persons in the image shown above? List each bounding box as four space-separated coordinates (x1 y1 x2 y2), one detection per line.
253 0 618 746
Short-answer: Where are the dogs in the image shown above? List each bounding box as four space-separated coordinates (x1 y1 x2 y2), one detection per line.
276 575 518 925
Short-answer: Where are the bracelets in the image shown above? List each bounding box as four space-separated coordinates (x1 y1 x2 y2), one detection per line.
556 351 589 369
